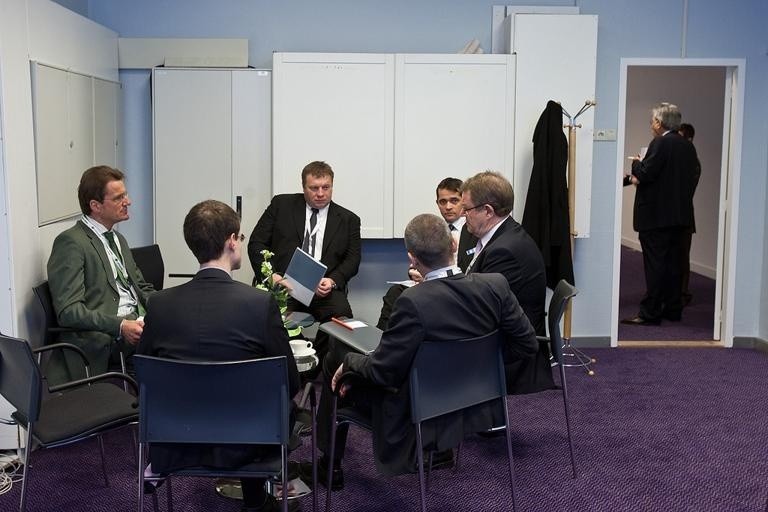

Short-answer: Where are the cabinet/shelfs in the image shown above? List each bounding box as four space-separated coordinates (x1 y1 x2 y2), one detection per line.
154 70 271 288
271 51 516 239
511 13 597 240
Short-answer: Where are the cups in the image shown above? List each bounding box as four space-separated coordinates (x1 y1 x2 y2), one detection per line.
288 339 313 354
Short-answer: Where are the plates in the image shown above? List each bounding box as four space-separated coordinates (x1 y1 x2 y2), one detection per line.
293 348 317 357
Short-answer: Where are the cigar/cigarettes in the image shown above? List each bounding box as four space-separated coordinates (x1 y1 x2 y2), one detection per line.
627 156 635 160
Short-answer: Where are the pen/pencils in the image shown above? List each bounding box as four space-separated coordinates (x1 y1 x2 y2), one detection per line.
627 157 634 159
331 317 353 330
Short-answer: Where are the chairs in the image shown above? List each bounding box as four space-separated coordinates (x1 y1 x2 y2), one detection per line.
132 354 315 511
535 279 578 479
326 329 517 511
1 244 164 510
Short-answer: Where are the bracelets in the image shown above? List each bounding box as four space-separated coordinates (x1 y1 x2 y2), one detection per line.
628 174 633 184
328 276 336 291
405 265 416 281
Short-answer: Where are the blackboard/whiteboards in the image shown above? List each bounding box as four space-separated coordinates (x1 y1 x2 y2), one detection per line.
30 61 121 228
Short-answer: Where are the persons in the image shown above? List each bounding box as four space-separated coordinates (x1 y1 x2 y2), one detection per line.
678 121 695 309
375 176 476 331
135 199 301 512
621 99 700 327
45 164 158 366
245 158 362 329
456 171 549 386
303 212 539 493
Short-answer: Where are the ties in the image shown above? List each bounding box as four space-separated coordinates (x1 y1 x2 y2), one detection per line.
303 207 319 257
104 232 129 289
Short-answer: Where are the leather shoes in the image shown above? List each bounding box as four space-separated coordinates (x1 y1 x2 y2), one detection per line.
275 460 344 491
410 449 454 471
623 315 656 326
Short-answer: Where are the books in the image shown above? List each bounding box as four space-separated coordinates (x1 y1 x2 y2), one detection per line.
280 247 327 310
383 279 419 289
639 146 648 161
318 316 386 356
